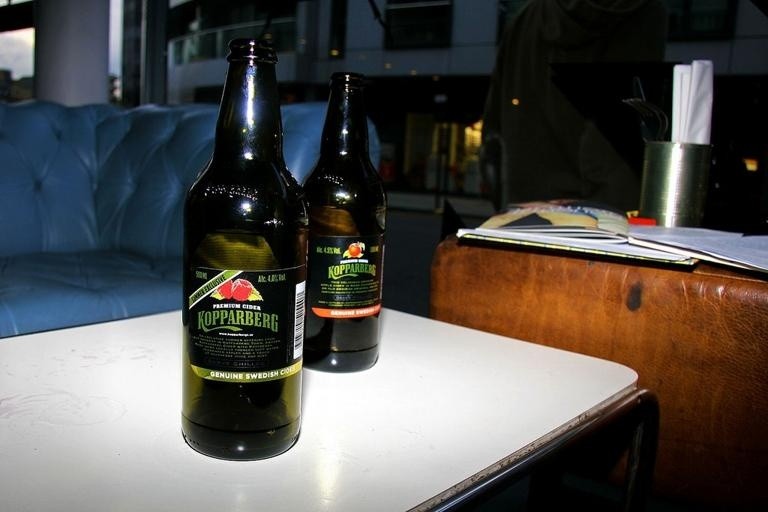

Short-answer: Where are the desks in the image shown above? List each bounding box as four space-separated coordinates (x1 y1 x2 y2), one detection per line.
430 235 767 512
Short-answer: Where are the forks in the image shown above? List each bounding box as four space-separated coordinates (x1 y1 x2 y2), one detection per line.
620 95 670 142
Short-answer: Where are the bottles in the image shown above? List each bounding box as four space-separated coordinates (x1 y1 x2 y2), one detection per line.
301 70 389 372
180 33 306 463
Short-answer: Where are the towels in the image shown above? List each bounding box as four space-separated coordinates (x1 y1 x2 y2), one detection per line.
671 58 714 146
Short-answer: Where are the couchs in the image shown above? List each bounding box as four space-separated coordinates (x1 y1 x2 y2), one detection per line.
1 99 381 341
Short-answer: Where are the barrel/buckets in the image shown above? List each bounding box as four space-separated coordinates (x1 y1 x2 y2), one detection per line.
639 141 716 228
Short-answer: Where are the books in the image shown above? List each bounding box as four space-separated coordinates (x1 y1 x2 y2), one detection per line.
455 201 768 274
